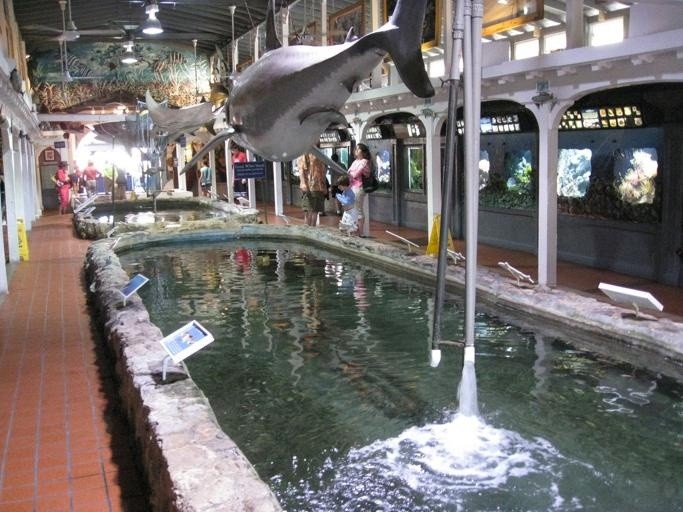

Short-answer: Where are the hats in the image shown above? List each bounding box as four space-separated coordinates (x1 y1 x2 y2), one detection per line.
58 161 69 167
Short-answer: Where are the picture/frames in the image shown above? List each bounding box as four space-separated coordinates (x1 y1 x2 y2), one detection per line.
289 1 544 64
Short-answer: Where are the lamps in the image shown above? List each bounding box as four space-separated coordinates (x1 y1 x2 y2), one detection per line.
121 1 163 65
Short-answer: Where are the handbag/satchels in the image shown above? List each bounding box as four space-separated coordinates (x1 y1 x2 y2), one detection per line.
50 175 57 185
362 164 379 194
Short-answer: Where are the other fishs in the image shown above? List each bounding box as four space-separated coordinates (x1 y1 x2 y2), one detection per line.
145 85 226 144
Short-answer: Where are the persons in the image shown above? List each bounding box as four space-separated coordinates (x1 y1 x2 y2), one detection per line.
329 153 349 217
331 174 359 236
49 160 103 215
345 143 374 237
267 252 372 389
197 158 211 199
296 140 327 229
230 144 246 201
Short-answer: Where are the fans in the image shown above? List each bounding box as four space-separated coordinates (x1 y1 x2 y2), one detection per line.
182 5 244 99
21 0 119 83
